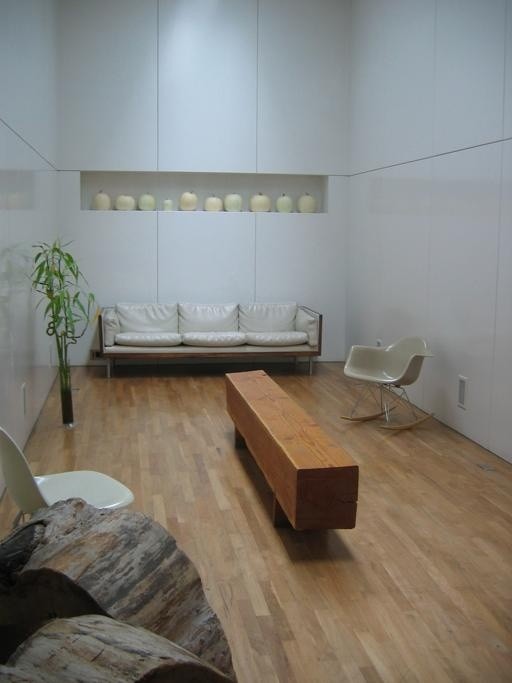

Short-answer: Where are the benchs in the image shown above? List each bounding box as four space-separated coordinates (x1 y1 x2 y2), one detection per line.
225 371 359 531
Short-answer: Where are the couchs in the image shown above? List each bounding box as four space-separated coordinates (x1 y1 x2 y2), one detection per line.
98 301 323 378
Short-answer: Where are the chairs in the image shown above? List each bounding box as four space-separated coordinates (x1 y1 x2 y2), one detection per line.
0 427 134 531
340 335 435 429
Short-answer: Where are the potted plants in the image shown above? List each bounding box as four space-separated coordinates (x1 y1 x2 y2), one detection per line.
27 237 96 428
